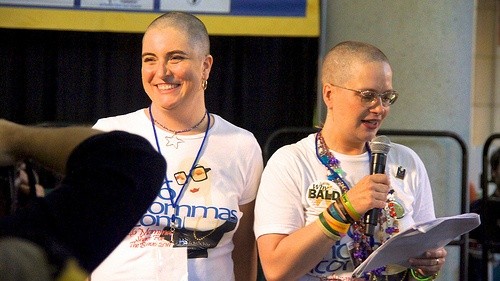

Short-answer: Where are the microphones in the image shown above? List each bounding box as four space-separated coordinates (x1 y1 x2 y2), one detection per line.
363 135 392 237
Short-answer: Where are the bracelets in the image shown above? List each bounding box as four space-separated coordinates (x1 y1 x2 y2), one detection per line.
316 190 362 241
410 266 439 281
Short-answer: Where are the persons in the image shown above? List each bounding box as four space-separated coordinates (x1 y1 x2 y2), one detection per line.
90 12 264 281
253 40 447 281
0 118 167 281
468 148 500 281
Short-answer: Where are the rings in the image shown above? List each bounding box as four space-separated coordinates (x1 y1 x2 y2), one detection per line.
436 258 439 265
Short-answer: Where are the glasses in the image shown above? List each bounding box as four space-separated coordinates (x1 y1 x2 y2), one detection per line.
330 84 399 107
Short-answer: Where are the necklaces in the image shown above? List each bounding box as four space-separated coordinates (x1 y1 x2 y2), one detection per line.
318 131 399 281
153 109 207 149
